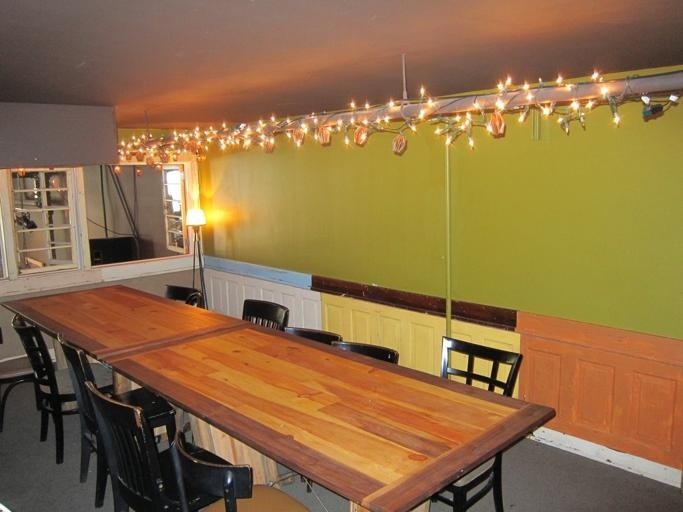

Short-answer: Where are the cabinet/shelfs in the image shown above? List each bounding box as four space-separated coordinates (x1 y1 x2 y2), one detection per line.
0 166 82 278
159 163 189 255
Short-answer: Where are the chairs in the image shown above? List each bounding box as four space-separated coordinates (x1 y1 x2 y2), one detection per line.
331 339 400 365
162 280 207 313
0 329 42 437
11 310 119 467
241 295 288 333
54 330 180 509
428 333 527 512
170 425 313 512
280 323 341 348
83 380 235 512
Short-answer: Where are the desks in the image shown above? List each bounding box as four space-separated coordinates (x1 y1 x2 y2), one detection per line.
100 318 559 512
1 284 249 362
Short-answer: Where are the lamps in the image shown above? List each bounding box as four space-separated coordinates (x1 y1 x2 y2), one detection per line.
183 205 209 312
